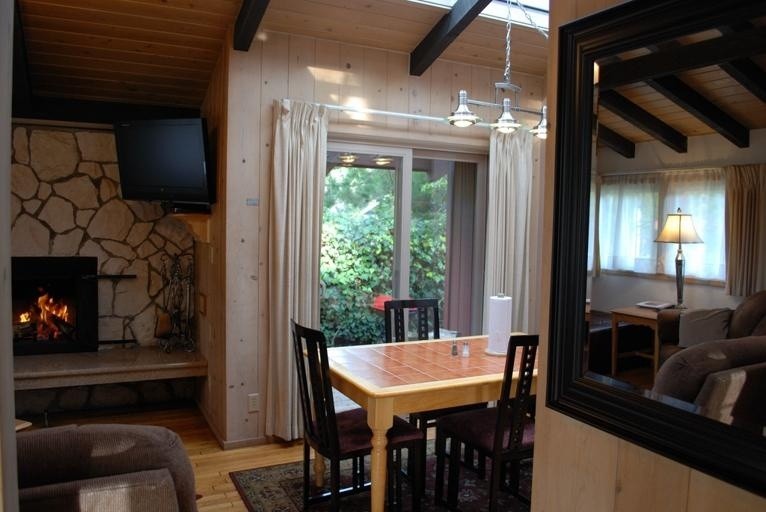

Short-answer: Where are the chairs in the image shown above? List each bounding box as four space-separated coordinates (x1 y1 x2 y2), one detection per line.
435 335 541 512
290 318 427 512
384 300 490 497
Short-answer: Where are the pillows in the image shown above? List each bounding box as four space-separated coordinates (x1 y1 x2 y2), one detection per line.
679 308 729 348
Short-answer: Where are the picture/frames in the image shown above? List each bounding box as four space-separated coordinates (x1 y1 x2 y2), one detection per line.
636 299 676 311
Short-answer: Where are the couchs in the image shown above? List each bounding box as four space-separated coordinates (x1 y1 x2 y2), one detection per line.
652 289 766 414
14 422 199 512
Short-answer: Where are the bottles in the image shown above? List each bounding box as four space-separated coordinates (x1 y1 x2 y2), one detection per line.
462 341 469 358
451 339 458 356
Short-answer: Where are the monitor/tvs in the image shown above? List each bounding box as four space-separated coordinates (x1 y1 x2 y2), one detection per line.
112 116 218 204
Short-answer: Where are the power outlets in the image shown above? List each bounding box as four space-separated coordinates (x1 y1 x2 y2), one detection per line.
247 392 262 413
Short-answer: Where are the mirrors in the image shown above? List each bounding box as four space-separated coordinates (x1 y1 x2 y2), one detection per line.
545 1 766 440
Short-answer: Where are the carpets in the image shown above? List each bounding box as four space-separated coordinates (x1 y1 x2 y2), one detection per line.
228 435 534 512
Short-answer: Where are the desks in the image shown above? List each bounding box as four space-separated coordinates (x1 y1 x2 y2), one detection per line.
301 332 540 512
12 343 208 391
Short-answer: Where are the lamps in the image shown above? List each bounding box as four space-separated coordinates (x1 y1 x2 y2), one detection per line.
653 207 705 311
372 157 394 166
447 0 546 141
339 155 358 163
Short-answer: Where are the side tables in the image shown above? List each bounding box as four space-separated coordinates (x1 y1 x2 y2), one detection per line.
610 304 689 386
